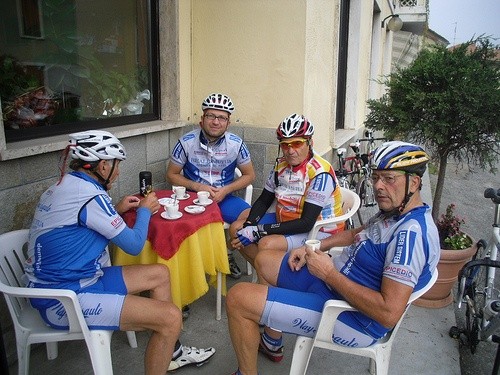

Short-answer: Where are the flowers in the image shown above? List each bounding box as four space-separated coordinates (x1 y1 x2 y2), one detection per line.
435 201 474 249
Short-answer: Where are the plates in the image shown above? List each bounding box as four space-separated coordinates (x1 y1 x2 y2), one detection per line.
161 212 183 220
158 197 180 206
184 205 206 214
170 194 190 200
192 198 213 206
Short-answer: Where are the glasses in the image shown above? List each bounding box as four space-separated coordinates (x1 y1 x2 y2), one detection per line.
280 138 308 150
369 172 415 184
204 113 230 122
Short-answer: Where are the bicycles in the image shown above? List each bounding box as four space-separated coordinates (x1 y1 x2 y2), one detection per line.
453 188 500 375
334 129 387 233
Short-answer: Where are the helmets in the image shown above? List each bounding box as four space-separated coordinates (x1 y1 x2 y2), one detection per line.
69 129 127 169
202 92 235 115
276 114 314 141
370 140 430 177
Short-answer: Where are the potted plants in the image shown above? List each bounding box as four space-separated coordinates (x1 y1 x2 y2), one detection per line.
362 32 500 309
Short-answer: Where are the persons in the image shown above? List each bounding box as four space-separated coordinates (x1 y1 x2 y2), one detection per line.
226 143 440 375
228 110 346 267
166 92 255 279
24 130 216 375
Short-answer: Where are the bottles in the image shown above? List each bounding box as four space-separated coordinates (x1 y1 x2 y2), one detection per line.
483 300 500 318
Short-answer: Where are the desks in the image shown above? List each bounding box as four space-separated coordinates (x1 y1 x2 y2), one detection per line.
109 190 231 321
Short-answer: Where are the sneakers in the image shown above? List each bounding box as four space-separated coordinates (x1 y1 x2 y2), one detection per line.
183 303 191 317
258 333 285 362
227 254 242 278
167 344 216 373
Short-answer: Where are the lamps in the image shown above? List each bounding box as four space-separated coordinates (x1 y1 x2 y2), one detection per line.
381 14 404 33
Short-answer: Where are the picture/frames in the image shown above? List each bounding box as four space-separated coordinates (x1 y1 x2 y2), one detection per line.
16 0 46 41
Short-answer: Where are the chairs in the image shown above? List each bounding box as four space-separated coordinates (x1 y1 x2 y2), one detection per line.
0 228 138 375
171 167 439 375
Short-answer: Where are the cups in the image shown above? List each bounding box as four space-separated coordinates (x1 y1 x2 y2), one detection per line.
305 239 320 252
164 204 179 218
173 186 186 198
197 191 209 203
